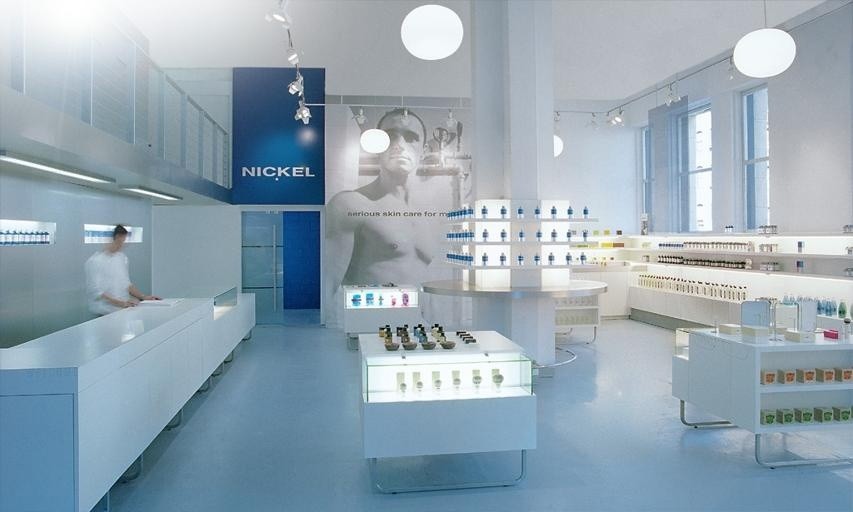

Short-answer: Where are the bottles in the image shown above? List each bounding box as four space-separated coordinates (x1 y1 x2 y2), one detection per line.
798 241 802 253
446 205 590 266
760 261 784 271
724 224 735 232
759 244 779 252
637 274 747 301
797 261 803 272
379 324 447 343
842 318 851 340
658 242 755 270
754 290 853 319
758 225 777 234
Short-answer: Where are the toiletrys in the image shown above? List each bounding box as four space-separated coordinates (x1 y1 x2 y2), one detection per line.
377 322 448 343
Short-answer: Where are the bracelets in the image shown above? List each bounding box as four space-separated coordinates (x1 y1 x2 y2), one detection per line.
140 294 145 301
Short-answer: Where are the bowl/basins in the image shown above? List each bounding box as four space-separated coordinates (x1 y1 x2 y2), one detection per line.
385 341 455 350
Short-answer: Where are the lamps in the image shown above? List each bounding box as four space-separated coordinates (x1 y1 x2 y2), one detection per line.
732 0 796 79
269 0 311 126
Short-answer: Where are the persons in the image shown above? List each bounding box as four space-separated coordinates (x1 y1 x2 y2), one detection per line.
84 224 162 318
327 108 433 292
